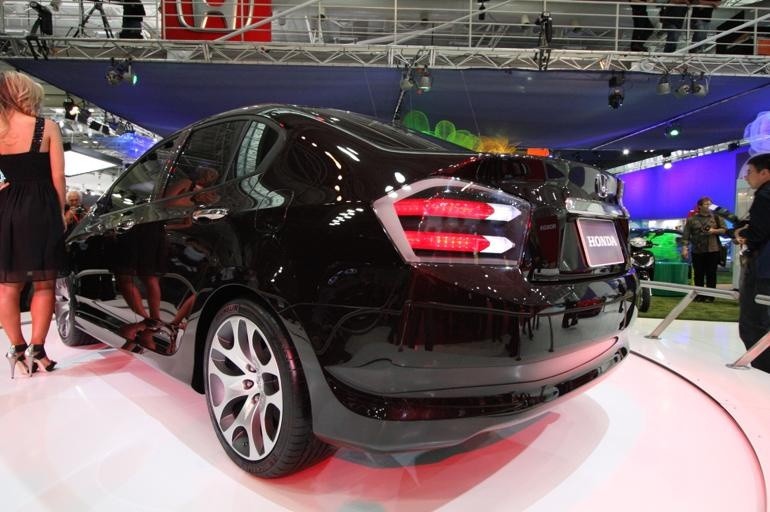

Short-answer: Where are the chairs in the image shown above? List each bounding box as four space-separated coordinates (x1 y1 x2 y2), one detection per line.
303 11 667 50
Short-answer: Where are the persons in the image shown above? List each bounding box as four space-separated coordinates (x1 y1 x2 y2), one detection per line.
113 164 220 363
64 190 88 228
688 0 723 57
656 2 691 57
680 197 728 306
629 1 655 53
116 0 147 40
1 68 72 380
733 152 770 372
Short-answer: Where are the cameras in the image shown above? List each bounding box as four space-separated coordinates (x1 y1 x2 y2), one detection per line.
75 207 84 214
709 203 749 238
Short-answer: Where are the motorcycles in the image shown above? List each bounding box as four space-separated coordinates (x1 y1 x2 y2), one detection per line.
628 228 664 311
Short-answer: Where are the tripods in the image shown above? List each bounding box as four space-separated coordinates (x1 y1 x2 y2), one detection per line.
73 2 114 38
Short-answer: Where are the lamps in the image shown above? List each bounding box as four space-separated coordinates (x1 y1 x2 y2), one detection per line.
59 88 138 140
100 54 720 96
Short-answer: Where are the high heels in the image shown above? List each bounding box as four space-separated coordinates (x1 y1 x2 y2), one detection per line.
23 343 59 378
5 342 39 380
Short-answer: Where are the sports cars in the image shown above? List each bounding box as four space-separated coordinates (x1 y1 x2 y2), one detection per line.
571 295 602 318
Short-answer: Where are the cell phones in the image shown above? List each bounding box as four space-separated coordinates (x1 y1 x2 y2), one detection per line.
705 225 711 231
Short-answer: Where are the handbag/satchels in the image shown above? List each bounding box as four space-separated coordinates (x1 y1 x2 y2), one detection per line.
719 241 728 269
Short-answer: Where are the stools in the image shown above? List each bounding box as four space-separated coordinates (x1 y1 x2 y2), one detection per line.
651 260 690 298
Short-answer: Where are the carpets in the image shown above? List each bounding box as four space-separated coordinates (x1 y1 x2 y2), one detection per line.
687 265 734 286
634 285 742 323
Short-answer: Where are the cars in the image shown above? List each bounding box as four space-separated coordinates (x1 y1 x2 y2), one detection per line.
629 227 684 263
57 102 645 480
